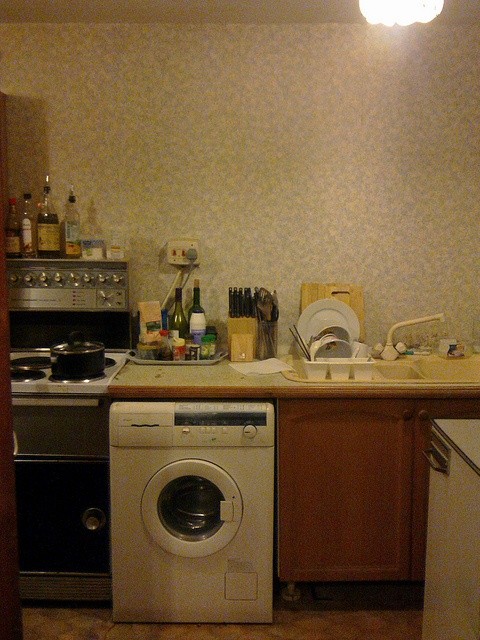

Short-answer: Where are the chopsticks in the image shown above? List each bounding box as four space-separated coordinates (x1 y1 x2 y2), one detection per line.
289 324 310 360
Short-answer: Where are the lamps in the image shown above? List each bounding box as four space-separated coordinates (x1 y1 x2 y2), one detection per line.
359 1 443 27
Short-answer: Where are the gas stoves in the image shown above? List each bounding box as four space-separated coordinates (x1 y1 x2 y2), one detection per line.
0 258 132 400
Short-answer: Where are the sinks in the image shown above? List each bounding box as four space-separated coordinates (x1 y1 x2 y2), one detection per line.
373 362 428 380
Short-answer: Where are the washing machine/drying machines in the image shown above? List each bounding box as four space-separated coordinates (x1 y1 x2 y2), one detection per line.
107 399 277 627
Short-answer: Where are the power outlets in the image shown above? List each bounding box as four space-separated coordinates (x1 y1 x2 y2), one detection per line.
167 240 200 267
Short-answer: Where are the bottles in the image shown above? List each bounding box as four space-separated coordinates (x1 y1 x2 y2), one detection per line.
206 334 217 356
189 344 201 360
36 186 60 259
171 337 186 361
4 198 22 258
20 193 37 258
60 196 81 259
200 336 211 360
168 288 188 339
188 279 207 345
182 334 194 360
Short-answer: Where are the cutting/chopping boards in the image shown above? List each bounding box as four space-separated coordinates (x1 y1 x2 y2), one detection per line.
300 282 367 345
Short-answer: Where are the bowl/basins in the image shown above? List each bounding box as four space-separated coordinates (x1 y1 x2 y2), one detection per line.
317 325 350 343
315 336 352 358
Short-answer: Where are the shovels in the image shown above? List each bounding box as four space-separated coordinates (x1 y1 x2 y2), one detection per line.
256 287 273 331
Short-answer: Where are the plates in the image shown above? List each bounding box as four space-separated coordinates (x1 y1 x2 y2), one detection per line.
295 298 360 354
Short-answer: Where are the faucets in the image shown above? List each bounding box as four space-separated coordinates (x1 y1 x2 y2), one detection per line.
380 311 446 362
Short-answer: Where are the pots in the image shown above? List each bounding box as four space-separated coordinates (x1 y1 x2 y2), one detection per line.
47 331 105 378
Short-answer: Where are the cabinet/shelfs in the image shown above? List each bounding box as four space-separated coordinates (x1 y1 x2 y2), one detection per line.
420 416 479 634
275 394 478 583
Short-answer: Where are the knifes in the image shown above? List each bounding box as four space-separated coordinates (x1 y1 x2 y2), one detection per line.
229 287 258 318
